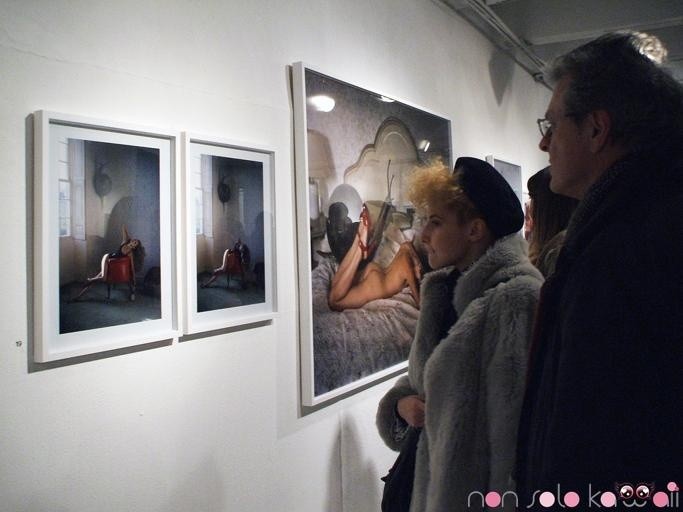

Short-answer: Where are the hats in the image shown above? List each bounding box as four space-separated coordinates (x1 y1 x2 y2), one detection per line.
452 158 524 238
528 165 560 196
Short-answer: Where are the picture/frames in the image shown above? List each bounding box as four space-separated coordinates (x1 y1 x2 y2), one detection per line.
290 59 454 406
487 154 524 239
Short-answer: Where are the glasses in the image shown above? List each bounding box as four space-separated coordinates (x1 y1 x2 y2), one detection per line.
537 111 588 139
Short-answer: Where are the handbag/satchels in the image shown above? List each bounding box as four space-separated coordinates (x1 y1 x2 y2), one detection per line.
381 448 416 512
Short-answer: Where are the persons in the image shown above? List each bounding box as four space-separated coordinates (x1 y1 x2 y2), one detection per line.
511 31 683 511
521 165 581 281
67 222 141 305
325 205 419 314
200 239 248 291
374 157 542 511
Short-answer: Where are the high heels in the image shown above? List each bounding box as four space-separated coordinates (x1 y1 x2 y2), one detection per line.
356 204 377 260
